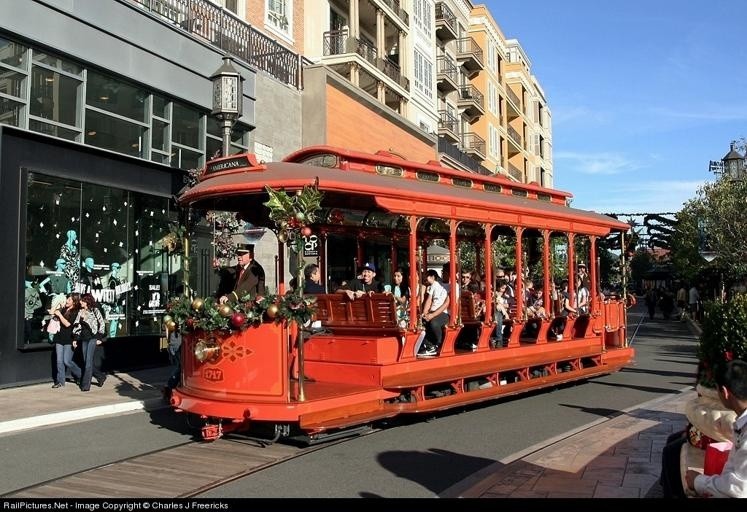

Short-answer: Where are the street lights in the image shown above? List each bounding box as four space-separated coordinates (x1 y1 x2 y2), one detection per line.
695 234 725 308
209 52 250 265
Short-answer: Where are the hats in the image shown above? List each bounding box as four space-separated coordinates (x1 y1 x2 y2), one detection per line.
361 261 376 271
234 242 256 254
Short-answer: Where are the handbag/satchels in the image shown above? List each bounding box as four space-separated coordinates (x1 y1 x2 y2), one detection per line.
97 323 108 339
46 319 61 336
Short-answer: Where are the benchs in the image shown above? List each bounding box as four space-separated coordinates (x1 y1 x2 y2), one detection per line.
303 292 602 365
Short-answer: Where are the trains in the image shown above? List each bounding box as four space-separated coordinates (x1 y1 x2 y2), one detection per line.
163 144 642 449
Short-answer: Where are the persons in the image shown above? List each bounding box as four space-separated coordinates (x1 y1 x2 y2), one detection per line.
106 262 124 338
645 280 747 320
60 229 81 290
684 358 746 498
24 280 43 343
159 285 195 406
71 294 107 392
80 257 106 320
46 294 82 389
219 243 265 305
39 258 71 343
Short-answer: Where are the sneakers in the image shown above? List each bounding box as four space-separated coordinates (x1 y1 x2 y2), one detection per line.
50 375 106 392
415 346 438 356
